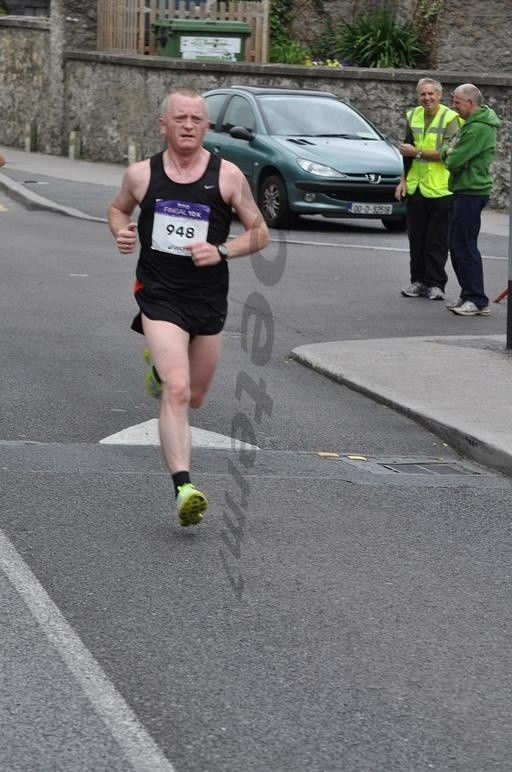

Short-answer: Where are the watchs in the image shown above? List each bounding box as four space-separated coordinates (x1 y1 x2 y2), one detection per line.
215 242 230 262
415 150 422 161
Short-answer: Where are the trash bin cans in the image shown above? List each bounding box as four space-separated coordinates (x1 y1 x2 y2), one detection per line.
151 18 252 62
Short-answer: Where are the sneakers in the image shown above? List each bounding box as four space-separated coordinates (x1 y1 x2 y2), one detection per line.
144 347 163 399
426 286 444 300
175 483 210 527
444 296 467 311
400 282 428 297
452 300 492 317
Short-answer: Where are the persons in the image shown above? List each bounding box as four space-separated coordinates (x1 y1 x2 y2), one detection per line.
394 76 465 300
439 81 498 322
105 87 271 528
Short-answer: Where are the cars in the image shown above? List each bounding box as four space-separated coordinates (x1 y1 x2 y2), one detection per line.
199 83 408 232
